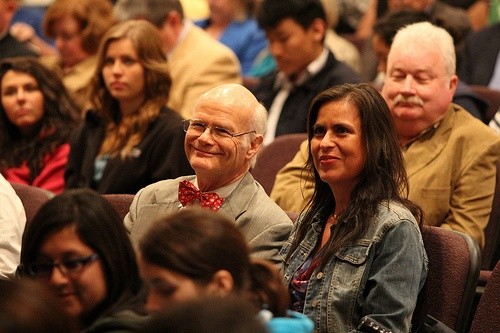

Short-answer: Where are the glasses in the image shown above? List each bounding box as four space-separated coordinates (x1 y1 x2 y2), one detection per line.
182 118 258 139
28 248 99 279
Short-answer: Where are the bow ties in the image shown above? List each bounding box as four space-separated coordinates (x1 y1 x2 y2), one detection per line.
177 179 226 211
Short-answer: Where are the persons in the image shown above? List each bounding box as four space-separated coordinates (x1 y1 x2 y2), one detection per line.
270 82 431 333
63 19 201 192
125 80 300 272
0 56 83 193
269 22 500 256
1 0 500 151
0 173 316 333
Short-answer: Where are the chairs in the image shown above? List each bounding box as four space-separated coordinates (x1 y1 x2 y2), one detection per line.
470 261 500 333
11 183 55 222
253 133 307 195
410 223 482 333
